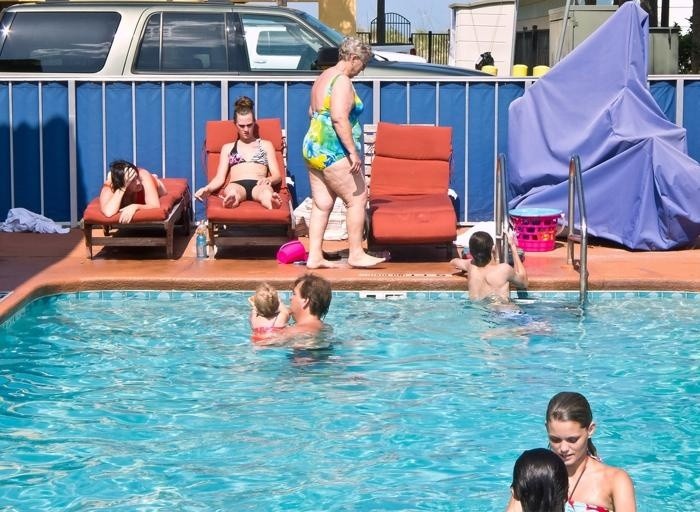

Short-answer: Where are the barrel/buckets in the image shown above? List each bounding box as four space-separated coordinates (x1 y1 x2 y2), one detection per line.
481 65 497 76
509 208 566 252
511 63 528 77
533 65 550 76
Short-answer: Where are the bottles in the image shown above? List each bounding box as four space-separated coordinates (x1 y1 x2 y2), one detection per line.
194 220 209 259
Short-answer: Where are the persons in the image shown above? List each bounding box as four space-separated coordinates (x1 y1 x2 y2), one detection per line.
252 271 336 366
302 34 388 271
249 281 291 345
508 446 568 512
505 390 636 512
450 229 531 309
196 95 285 209
99 158 166 225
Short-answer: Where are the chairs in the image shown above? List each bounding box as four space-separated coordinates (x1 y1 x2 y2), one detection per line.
200 118 295 261
362 121 457 260
82 176 191 259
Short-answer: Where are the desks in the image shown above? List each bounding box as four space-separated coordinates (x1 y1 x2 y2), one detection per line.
291 197 360 240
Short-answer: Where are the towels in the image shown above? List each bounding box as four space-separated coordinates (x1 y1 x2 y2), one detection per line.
452 220 510 247
0 207 71 235
